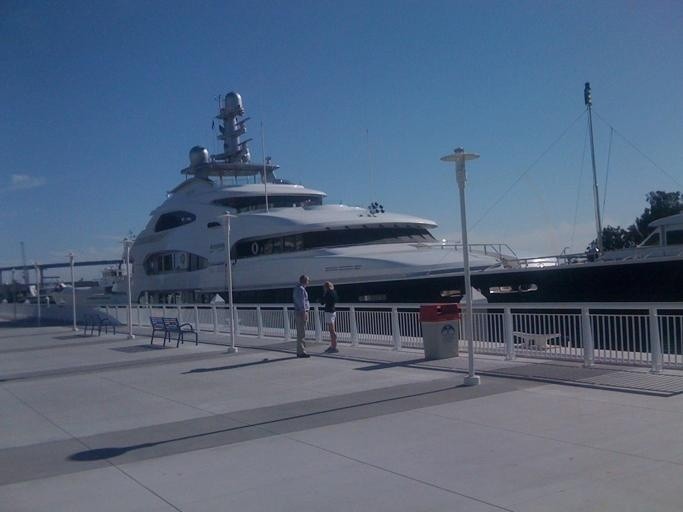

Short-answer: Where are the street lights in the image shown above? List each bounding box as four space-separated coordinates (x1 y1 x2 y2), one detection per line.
439 146 482 387
217 210 239 353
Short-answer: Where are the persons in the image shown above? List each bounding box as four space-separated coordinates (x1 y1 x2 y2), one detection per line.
318 280 339 353
292 275 311 357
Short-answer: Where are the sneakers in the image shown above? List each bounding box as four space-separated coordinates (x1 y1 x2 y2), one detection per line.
325 347 338 353
297 353 310 358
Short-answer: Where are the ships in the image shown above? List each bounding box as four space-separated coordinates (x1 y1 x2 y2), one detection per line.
52 89 682 341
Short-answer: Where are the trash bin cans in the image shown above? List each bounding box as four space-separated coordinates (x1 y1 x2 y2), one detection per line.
419 304 459 360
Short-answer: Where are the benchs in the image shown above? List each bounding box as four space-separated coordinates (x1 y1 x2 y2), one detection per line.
82 312 118 337
148 315 199 349
